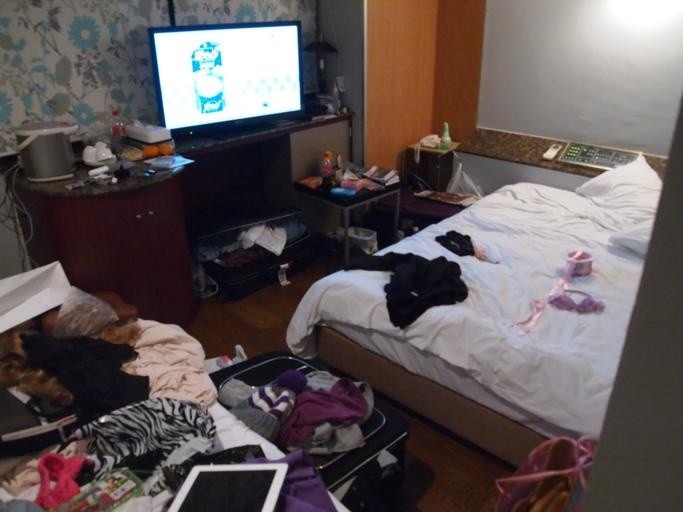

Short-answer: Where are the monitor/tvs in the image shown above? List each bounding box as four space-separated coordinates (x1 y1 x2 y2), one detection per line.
146 19 308 142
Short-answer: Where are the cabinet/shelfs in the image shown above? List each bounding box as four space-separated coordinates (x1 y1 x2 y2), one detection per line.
405 140 460 192
9 177 203 330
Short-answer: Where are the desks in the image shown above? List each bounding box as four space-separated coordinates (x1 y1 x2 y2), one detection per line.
288 161 401 266
7 104 354 340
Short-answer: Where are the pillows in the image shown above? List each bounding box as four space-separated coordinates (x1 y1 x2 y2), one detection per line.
575 152 664 256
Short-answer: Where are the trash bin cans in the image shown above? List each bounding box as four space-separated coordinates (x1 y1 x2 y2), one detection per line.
348 227 378 265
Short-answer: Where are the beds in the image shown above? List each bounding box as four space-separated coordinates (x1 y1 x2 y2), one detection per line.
286 182 663 469
0 399 352 512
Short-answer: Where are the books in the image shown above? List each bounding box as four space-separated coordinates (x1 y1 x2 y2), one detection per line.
363 164 400 186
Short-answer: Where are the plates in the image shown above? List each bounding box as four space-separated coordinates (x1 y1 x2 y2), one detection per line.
85 157 117 167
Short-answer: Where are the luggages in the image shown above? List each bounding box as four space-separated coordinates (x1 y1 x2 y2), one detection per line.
209 349 411 512
188 193 317 302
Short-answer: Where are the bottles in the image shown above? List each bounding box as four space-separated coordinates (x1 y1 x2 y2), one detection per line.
332 153 342 186
439 122 450 149
319 151 332 175
111 111 128 155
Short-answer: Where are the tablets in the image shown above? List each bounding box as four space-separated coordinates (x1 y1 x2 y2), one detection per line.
167 462 289 512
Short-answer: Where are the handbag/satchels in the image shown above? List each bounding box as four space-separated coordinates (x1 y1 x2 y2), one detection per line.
0 260 72 334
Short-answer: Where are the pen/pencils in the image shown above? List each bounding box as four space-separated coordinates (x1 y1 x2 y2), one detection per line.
48 468 142 512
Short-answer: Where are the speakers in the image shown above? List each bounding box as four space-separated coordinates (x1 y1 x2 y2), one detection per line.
405 149 454 192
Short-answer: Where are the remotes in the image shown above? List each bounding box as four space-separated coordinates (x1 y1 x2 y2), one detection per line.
541 143 562 161
330 187 356 197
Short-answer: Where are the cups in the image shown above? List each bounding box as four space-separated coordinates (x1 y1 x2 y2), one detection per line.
81 142 112 162
